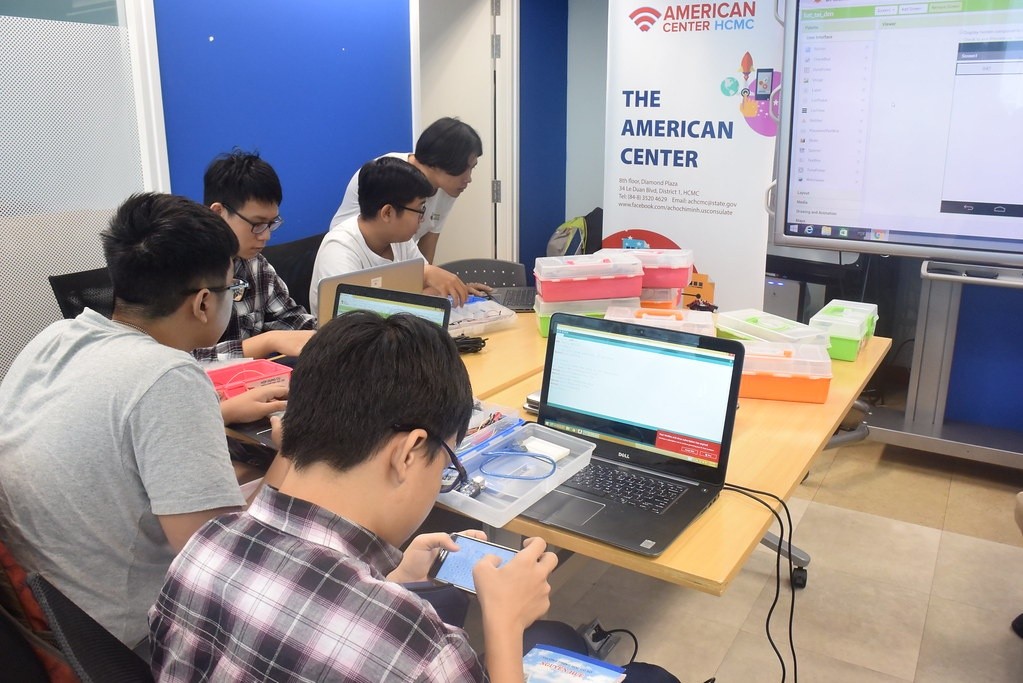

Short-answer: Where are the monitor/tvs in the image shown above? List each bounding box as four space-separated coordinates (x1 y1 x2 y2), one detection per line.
774 0 1023 268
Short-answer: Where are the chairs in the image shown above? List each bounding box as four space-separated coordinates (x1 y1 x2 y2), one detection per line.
759 398 873 588
25 570 158 683
48 268 115 321
438 258 527 288
1012 491 1023 639
261 232 328 314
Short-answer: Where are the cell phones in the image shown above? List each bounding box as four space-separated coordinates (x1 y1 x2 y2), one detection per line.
427 531 521 594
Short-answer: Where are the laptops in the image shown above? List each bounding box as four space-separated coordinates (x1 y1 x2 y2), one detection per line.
478 312 745 558
273 257 425 372
477 228 584 310
265 282 451 423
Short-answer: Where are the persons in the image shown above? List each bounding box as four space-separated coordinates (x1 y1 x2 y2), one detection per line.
146 310 682 683
309 156 468 329
190 151 317 363
0 191 289 649
329 117 493 296
1011 492 1023 640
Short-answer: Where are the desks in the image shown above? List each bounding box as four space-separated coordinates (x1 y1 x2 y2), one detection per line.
225 311 895 599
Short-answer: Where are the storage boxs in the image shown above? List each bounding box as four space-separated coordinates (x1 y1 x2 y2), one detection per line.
532 253 646 303
595 247 695 289
435 394 596 530
532 294 641 339
448 299 519 339
605 305 717 337
728 339 831 404
639 287 678 310
204 358 294 402
809 299 880 361
715 308 810 341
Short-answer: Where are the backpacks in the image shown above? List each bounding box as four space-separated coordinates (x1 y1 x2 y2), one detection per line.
546 207 604 257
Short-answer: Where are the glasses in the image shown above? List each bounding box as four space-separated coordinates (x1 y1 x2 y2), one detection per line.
393 420 468 492
380 200 427 220
220 201 283 234
182 278 251 302
